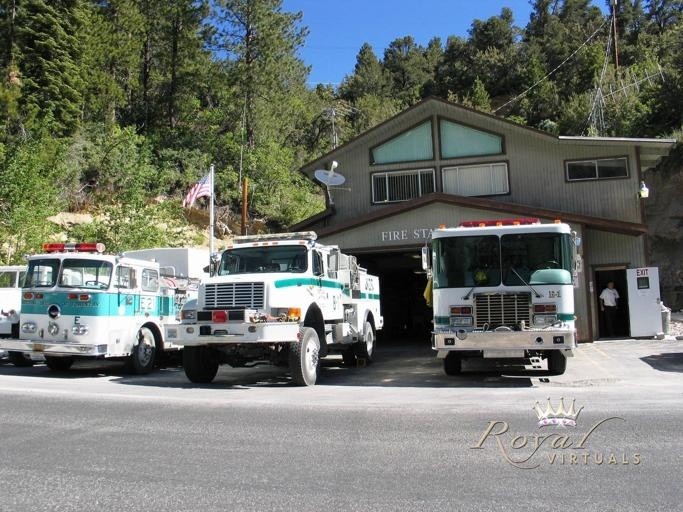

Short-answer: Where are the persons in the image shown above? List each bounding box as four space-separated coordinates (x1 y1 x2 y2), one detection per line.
597 281 620 338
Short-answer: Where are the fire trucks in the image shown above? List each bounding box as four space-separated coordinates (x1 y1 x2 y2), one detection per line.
175 230 384 386
0 242 200 374
421 216 583 375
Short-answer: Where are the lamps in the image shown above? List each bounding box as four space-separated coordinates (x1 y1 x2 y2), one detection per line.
637 179 649 199
314 160 347 187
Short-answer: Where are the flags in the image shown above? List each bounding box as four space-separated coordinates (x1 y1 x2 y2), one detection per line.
181 168 213 211
